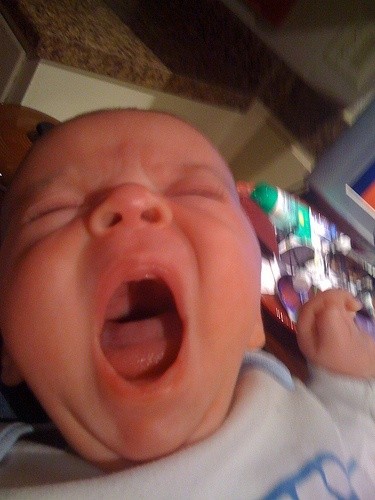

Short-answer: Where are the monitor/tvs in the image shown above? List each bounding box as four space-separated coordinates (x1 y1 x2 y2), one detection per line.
303 92 375 253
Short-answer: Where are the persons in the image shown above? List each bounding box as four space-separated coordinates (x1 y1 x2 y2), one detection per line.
0 106 375 500
